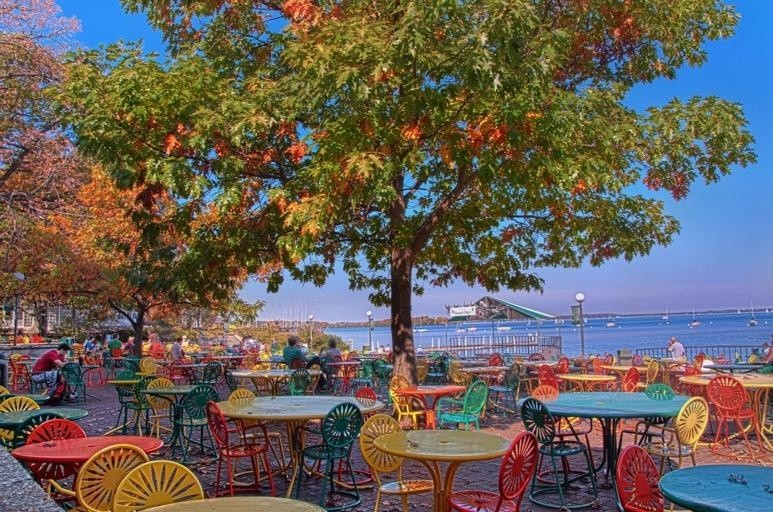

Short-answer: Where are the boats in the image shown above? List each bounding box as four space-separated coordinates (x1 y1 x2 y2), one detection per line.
603 319 621 329
459 326 516 335
687 318 705 328
741 316 761 327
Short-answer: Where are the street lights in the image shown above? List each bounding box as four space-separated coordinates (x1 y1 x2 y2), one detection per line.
573 287 589 360
10 269 23 345
305 313 319 356
364 309 376 353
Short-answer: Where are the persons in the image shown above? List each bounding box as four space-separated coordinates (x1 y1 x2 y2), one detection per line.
238 335 278 357
81 332 134 356
32 344 80 401
146 334 200 362
588 350 616 364
282 332 341 389
666 335 684 359
